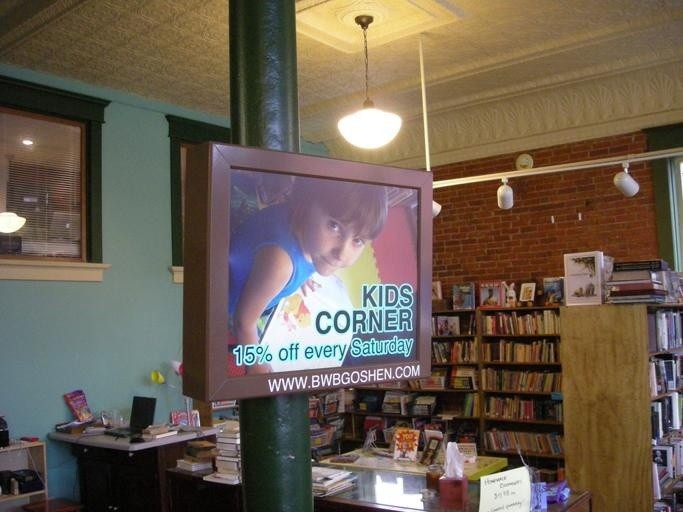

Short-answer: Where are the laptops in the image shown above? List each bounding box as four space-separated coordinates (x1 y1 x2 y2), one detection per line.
102 395 157 438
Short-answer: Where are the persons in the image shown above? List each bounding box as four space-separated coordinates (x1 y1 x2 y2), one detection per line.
228 175 387 375
483 289 497 305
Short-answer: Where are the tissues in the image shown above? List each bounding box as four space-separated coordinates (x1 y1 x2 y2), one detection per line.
437 441 469 510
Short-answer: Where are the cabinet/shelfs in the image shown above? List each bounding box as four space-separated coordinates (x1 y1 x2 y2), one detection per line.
0 441 49 511
338 308 565 486
560 303 683 512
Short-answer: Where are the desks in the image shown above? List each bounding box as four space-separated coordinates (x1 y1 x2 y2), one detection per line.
317 448 507 481
48 426 225 512
161 463 593 512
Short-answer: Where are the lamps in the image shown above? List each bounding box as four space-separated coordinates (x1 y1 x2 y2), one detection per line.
0 154 26 234
496 179 514 210
614 164 639 197
337 16 402 149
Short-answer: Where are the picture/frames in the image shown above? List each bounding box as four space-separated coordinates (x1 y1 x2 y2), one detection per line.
431 200 441 219
186 145 434 400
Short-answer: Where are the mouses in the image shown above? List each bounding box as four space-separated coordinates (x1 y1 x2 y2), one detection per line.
130 436 147 444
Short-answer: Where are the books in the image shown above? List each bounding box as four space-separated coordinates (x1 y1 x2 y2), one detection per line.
431 312 475 336
176 440 215 472
484 396 563 423
649 309 683 511
202 421 241 485
419 367 478 391
482 339 560 364
430 337 478 364
481 368 561 392
483 309 560 335
312 466 358 498
484 429 563 456
142 424 178 440
451 282 475 310
464 394 480 417
606 259 683 304
